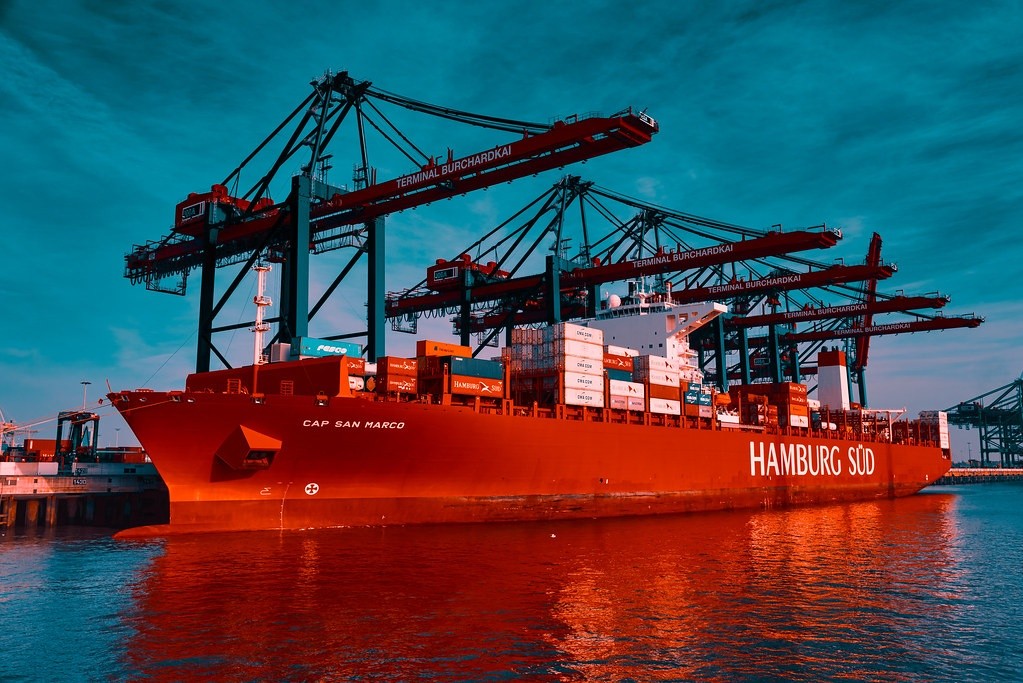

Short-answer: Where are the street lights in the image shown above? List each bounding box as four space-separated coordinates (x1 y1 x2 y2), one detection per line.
80 382 92 411
115 428 121 447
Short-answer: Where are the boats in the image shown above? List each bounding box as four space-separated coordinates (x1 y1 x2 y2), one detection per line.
104 69 987 540
